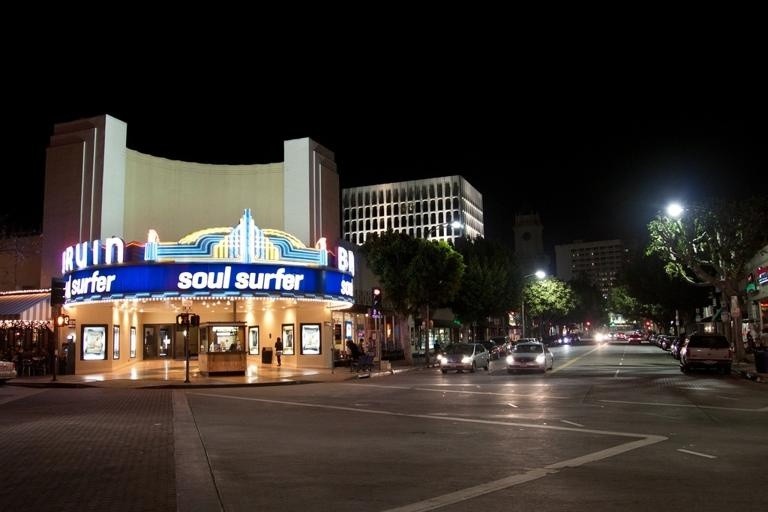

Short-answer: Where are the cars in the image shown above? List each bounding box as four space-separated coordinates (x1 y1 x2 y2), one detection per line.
440 342 490 374
506 340 554 373
436 345 454 366
0 361 17 383
477 332 685 361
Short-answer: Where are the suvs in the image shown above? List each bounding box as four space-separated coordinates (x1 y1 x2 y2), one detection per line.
677 333 735 375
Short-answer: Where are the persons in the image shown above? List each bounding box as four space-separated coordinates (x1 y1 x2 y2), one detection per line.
274 336 284 366
345 337 376 372
434 340 440 354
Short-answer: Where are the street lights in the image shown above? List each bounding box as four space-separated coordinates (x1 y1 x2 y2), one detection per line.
665 201 746 364
521 269 546 338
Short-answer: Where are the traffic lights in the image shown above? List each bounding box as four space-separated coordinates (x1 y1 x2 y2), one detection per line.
584 325 589 331
585 317 591 327
611 322 615 332
646 322 651 329
57 315 69 327
372 287 382 310
176 314 188 327
191 315 199 326
645 317 650 326
51 277 66 307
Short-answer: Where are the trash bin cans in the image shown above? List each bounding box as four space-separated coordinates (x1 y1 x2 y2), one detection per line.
754 346 768 374
263 347 272 364
